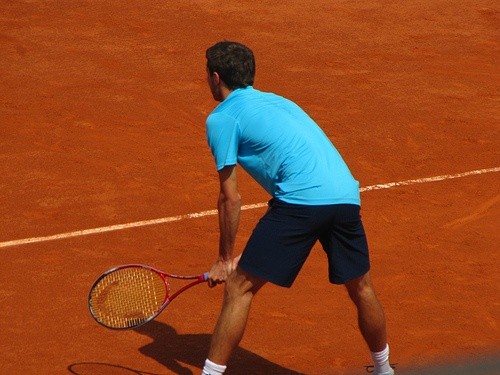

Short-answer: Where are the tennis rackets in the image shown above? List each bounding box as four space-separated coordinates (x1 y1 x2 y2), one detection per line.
88 264 209 331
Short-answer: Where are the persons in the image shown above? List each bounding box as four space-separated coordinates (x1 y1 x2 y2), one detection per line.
197 39 399 375
